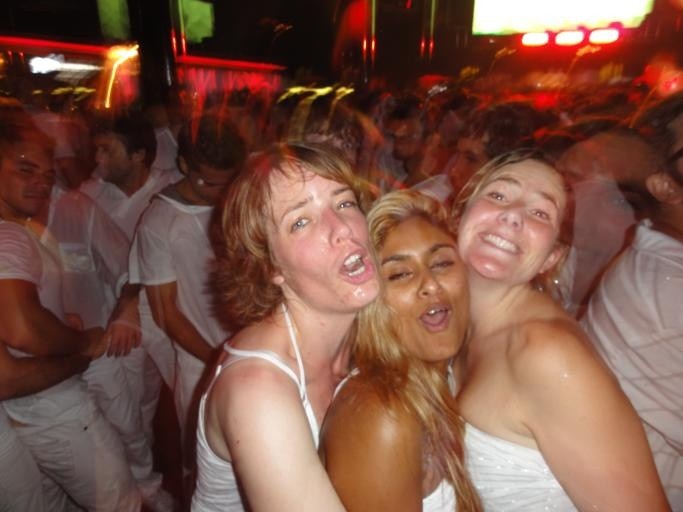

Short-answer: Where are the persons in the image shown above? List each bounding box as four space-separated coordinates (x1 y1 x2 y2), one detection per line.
0 48 682 512
